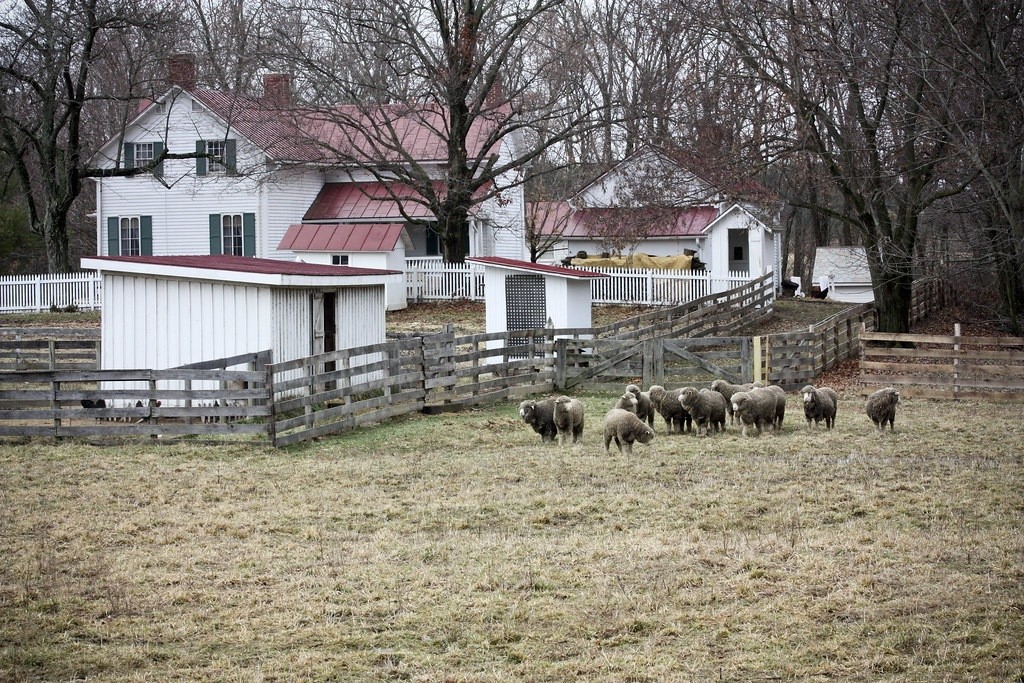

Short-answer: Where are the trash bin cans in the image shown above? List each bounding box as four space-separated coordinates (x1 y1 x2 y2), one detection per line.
781 280 800 297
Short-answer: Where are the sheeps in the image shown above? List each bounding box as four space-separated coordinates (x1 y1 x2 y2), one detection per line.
866 387 902 433
519 395 584 448
798 384 839 430
602 379 786 454
